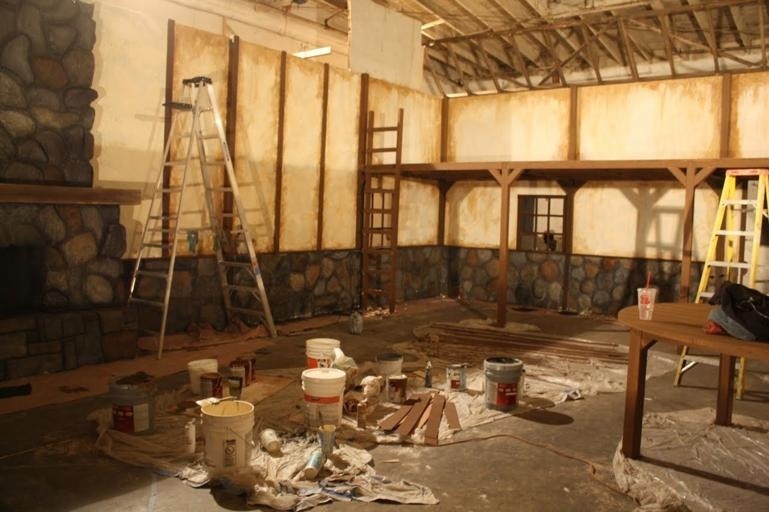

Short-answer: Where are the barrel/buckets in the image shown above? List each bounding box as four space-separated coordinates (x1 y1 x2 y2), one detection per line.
306 338 340 368
484 357 523 410
186 359 220 394
109 378 157 434
375 349 403 378
445 363 467 390
200 401 257 473
301 369 346 432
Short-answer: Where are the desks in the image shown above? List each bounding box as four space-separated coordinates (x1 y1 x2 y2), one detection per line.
618 303 769 458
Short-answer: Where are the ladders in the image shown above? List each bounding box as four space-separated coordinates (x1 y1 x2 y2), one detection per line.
361 109 405 314
126 76 277 359
674 169 769 401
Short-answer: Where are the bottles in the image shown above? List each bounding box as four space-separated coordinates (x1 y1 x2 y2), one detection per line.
424 361 433 387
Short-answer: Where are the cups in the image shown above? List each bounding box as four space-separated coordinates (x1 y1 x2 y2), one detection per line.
637 288 657 320
304 450 327 479
318 424 336 457
260 428 280 452
